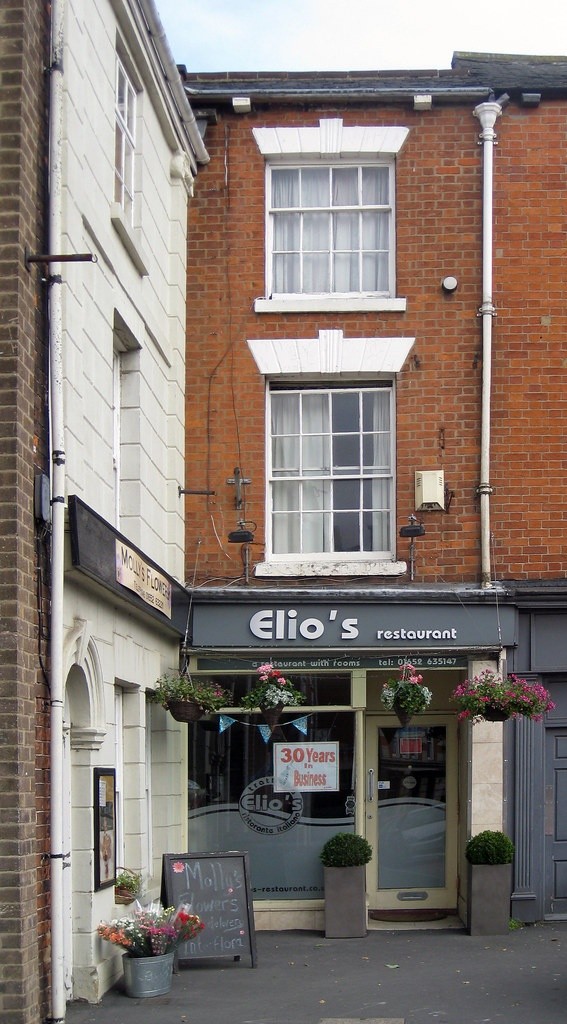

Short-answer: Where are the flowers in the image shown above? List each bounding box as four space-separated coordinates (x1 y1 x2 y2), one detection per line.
241 665 307 715
97 900 208 957
448 668 555 726
380 663 433 713
145 670 233 714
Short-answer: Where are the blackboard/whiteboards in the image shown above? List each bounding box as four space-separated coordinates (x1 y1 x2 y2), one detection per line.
166 852 257 959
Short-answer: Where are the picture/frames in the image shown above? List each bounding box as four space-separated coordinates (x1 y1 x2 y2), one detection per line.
93 769 117 891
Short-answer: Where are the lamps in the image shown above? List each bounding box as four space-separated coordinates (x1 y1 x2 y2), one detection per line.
398 514 425 581
227 518 257 583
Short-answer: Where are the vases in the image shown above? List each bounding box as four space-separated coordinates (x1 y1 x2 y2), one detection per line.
122 950 175 999
166 701 206 723
478 705 511 722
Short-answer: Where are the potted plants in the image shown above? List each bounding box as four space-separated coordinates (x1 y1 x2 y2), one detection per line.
464 829 514 935
115 871 143 904
320 832 372 938
